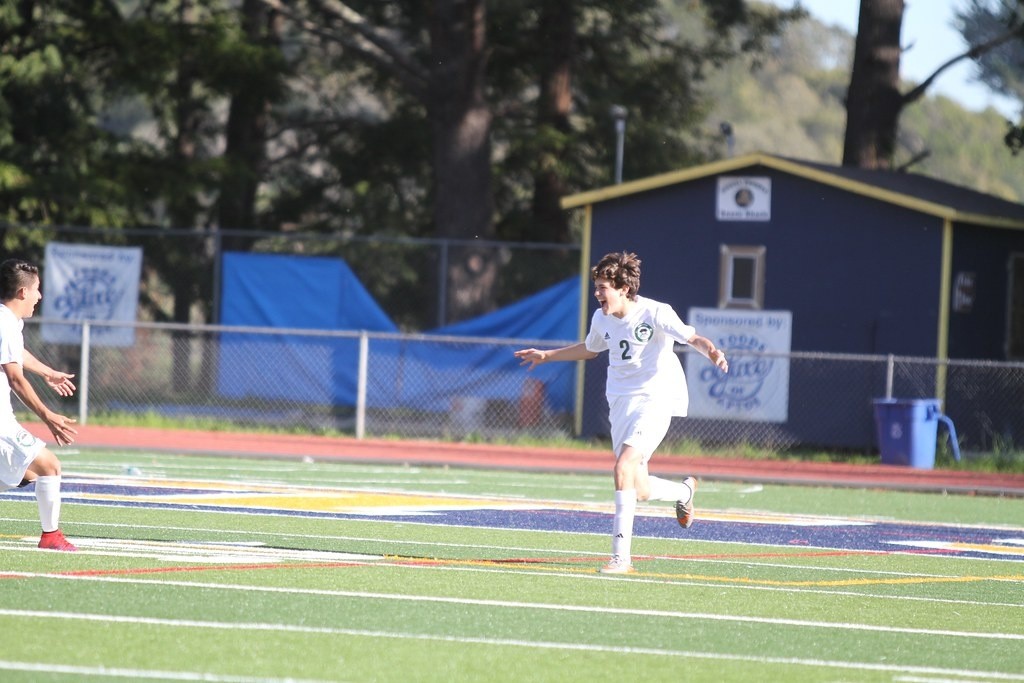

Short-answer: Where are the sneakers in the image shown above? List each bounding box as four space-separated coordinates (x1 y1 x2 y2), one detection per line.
673 476 697 528
600 555 634 574
38 529 76 552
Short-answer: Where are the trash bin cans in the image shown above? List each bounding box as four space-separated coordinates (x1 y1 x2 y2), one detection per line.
872 398 961 469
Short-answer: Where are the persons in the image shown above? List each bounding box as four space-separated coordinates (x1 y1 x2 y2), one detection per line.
514 252 729 573
0 258 83 553
